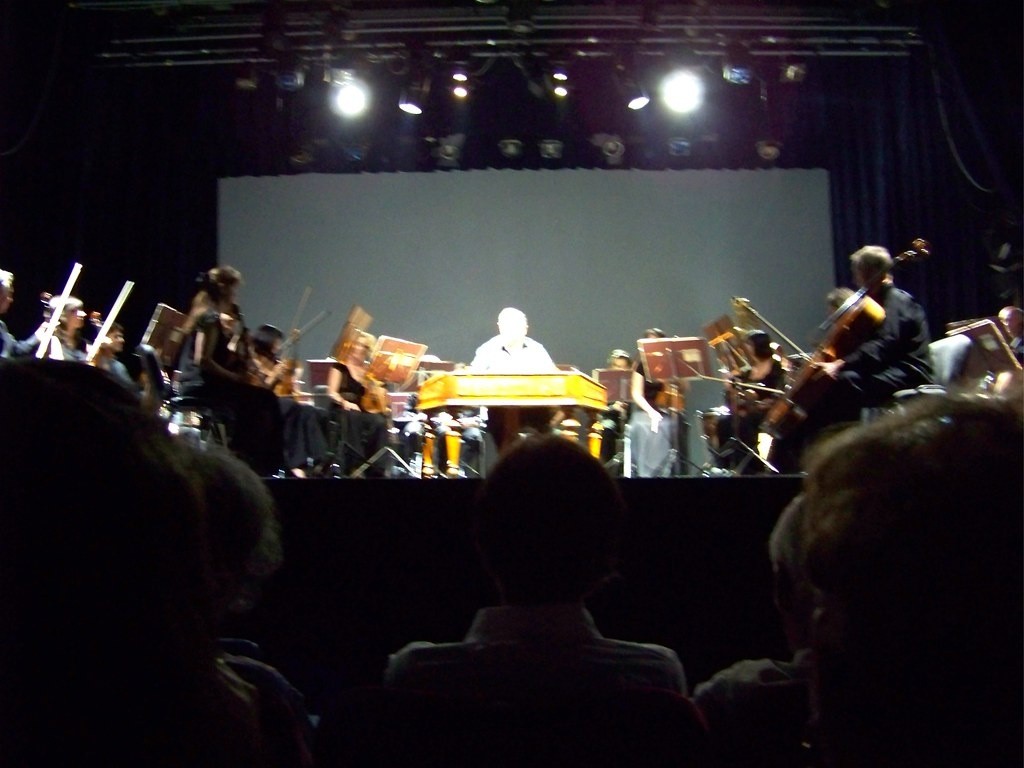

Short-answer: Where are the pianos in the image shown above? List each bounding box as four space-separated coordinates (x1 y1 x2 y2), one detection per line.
414 366 610 480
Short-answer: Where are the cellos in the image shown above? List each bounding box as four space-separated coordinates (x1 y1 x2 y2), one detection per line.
761 236 933 441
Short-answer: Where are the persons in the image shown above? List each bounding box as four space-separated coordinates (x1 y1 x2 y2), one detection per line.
998 307 1024 364
384 438 691 698
46 295 95 362
783 245 934 472
471 307 559 450
89 321 132 383
250 321 329 474
0 357 317 767
631 328 692 478
328 331 392 477
180 264 294 475
607 348 633 370
696 394 1024 767
0 269 52 359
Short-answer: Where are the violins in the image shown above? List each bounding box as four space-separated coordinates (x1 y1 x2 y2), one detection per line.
272 328 303 399
360 351 387 414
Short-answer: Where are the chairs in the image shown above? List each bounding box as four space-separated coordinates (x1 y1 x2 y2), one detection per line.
138 343 226 448
868 333 973 429
